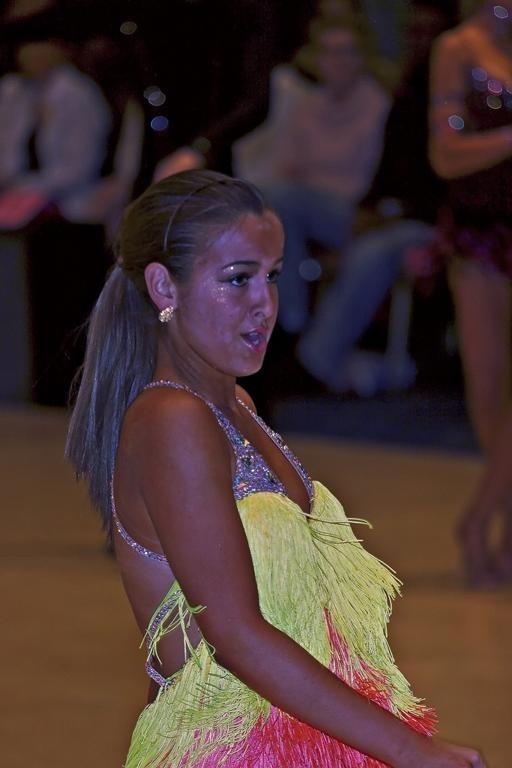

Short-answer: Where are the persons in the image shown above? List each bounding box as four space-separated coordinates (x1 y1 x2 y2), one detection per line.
0 1 512 594
59 166 488 768
